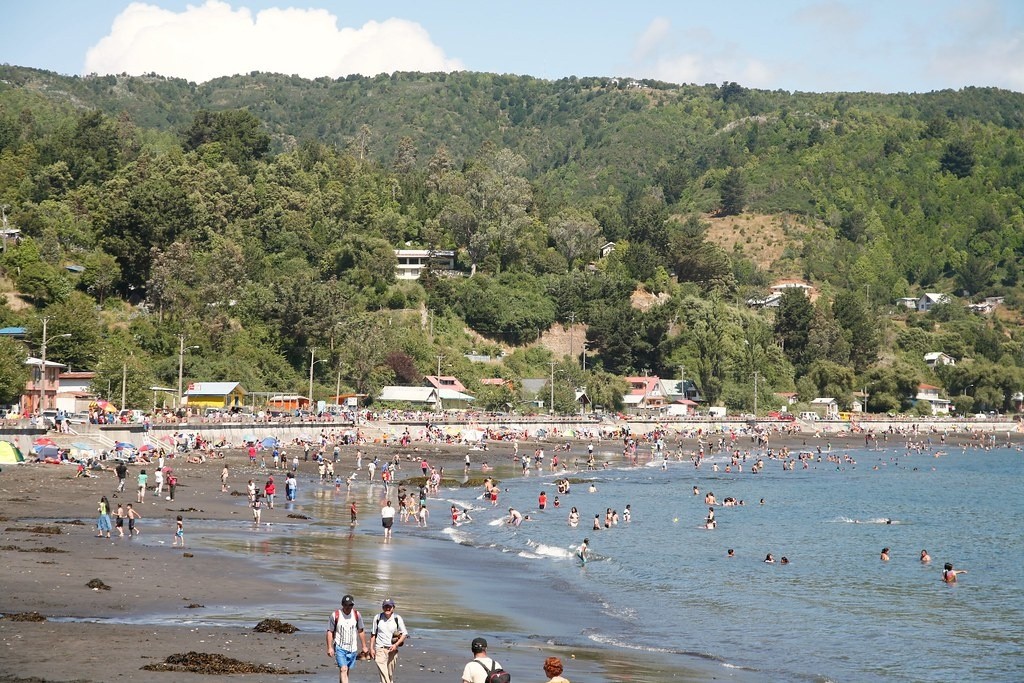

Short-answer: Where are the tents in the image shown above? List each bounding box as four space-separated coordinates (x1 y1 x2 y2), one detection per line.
0 440 24 463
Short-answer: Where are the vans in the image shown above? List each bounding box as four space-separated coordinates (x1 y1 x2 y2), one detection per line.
801 412 820 421
838 412 851 421
118 409 144 424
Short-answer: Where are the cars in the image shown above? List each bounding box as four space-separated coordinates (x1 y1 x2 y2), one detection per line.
204 406 314 422
919 415 939 421
43 408 89 428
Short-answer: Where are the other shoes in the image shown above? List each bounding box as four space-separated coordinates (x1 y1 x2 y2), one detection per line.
95 534 103 537
356 652 365 661
105 535 111 538
393 632 402 639
392 637 404 647
365 651 370 660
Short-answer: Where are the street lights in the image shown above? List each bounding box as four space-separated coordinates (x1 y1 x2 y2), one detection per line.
309 360 327 410
551 370 563 410
865 383 874 414
40 334 72 415
178 346 200 409
436 364 452 415
965 385 974 418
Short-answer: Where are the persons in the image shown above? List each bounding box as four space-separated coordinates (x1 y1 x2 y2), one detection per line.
920 550 931 560
764 553 775 562
461 638 502 683
881 548 890 560
326 594 368 683
543 656 570 683
941 563 966 582
577 537 590 562
370 598 408 683
781 557 788 563
727 549 734 556
0 404 1024 546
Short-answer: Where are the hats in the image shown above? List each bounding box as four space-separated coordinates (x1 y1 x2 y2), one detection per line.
382 598 394 607
342 594 354 606
472 637 487 648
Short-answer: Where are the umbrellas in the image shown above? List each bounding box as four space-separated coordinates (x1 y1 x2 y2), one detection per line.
139 445 153 451
113 443 134 448
96 399 117 412
33 438 57 445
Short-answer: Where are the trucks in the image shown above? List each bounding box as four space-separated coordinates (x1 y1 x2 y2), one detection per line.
708 407 726 419
659 404 687 417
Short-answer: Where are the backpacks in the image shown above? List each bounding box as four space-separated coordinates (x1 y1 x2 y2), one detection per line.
474 660 511 683
